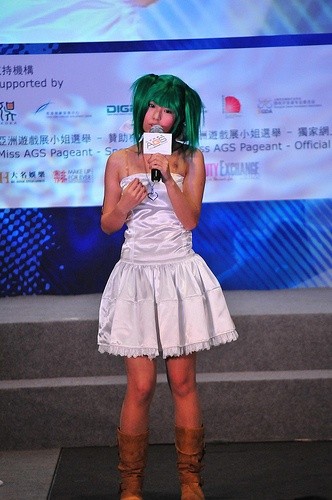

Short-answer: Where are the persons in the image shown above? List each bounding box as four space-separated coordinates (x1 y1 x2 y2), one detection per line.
96 73 239 500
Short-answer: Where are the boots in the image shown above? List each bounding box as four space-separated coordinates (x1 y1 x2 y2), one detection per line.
116 426 150 500
173 423 208 500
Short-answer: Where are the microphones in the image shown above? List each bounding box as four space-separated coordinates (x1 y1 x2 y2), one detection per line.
150 124 165 183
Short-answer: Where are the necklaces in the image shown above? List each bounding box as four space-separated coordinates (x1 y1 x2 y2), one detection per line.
142 156 161 202
